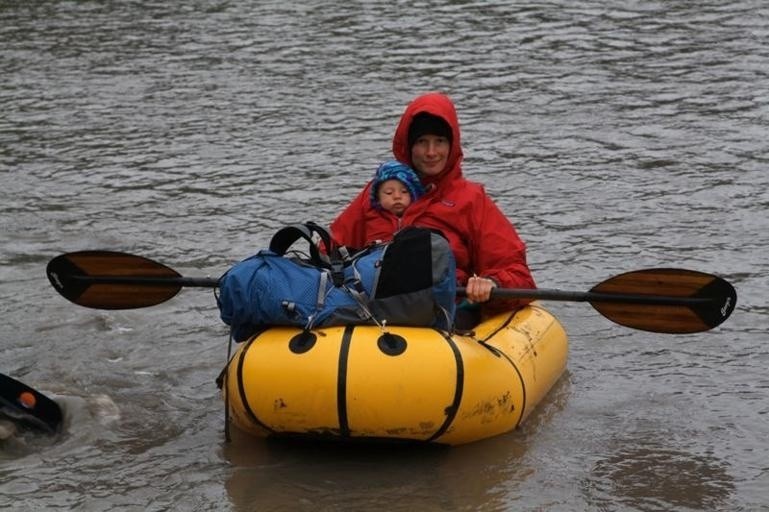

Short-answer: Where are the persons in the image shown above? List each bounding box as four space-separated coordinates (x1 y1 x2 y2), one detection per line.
347 160 470 311
318 92 538 334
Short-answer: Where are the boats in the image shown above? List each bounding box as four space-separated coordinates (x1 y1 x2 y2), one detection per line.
216 300 572 452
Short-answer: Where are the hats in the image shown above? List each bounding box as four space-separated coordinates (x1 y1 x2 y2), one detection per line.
407 113 451 158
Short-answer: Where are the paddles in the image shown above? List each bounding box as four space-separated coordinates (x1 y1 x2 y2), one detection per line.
0 372 62 437
46 250 737 333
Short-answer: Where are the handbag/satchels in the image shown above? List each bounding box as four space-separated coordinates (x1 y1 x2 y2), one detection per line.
215 219 458 331
